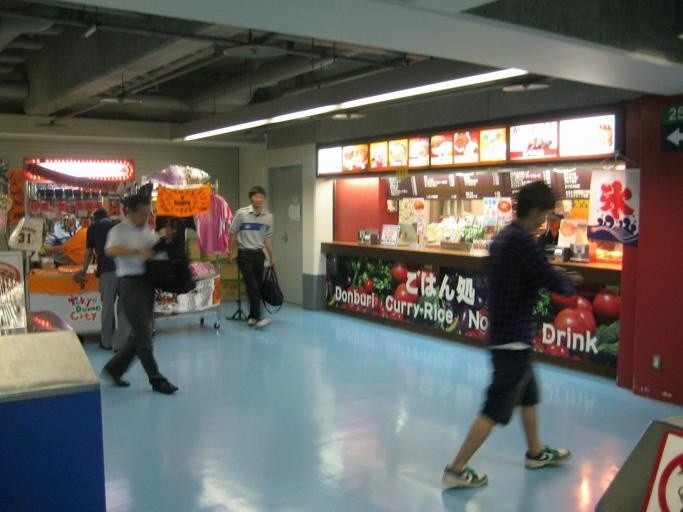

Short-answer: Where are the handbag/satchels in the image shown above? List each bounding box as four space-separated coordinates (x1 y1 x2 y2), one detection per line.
146 232 196 294
260 266 283 307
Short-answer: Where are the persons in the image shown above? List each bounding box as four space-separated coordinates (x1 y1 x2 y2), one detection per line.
44 213 82 265
226 185 276 329
72 207 132 351
537 208 564 246
440 180 575 489
100 194 179 394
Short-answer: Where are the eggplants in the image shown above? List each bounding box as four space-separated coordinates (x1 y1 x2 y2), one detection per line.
445 290 484 332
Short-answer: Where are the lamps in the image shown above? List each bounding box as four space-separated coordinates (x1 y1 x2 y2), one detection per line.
35 186 101 200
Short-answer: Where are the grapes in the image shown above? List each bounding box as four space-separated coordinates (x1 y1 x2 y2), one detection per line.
532 330 569 358
340 279 403 320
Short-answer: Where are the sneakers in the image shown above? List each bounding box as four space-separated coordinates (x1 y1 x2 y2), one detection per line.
441 465 488 489
101 363 131 387
246 317 270 329
526 447 572 470
153 380 179 394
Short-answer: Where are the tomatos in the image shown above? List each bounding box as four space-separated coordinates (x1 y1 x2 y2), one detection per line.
553 291 620 338
392 262 435 302
